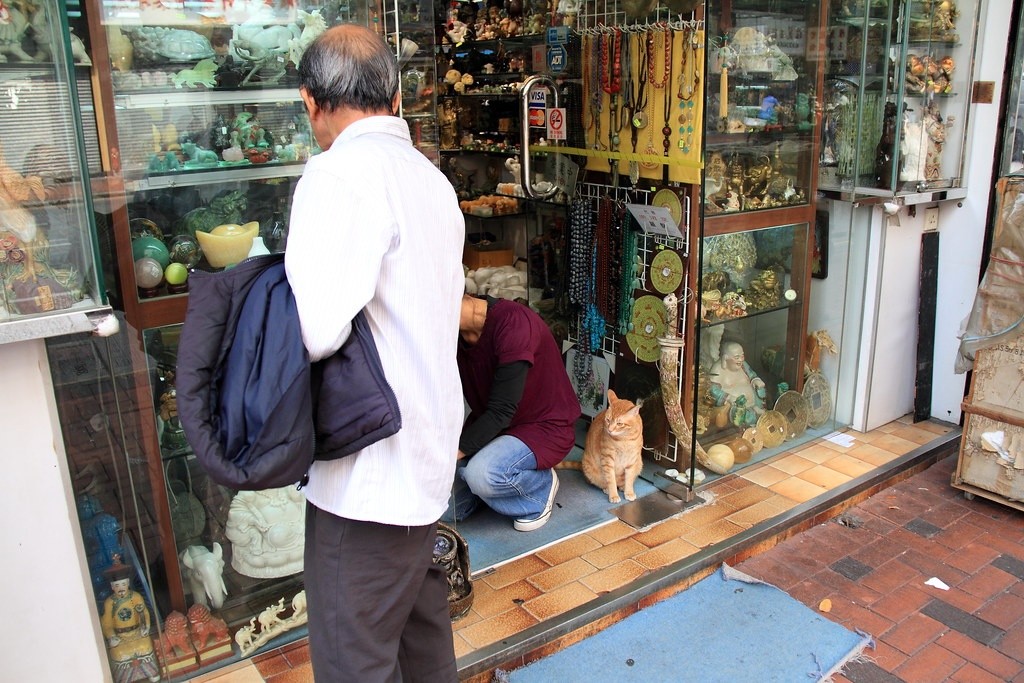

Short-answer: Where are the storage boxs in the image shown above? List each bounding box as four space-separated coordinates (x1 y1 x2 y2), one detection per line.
463 244 513 272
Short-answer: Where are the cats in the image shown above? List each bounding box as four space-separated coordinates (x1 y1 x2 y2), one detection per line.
552 388 644 504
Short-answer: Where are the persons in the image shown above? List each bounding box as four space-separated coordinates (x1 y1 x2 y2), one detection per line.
874 101 951 189
130 52 302 228
440 286 582 531
225 485 306 578
283 24 465 683
98 564 161 683
22 144 74 266
708 341 767 425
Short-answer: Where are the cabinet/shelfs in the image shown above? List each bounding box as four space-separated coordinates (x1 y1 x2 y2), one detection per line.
788 0 983 200
0 0 116 343
78 0 380 648
432 33 582 206
584 0 830 472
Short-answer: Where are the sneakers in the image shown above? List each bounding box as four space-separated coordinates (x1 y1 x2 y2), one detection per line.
456 496 480 522
514 466 559 532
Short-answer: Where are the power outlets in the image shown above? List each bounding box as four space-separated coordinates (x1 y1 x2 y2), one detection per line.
924 207 939 230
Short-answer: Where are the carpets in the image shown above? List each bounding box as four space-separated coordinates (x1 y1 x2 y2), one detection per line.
488 560 876 683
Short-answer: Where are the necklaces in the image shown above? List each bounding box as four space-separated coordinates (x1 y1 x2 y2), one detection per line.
570 199 638 381
558 22 699 187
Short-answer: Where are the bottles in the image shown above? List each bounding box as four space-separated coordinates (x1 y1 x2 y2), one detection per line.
249 237 271 258
